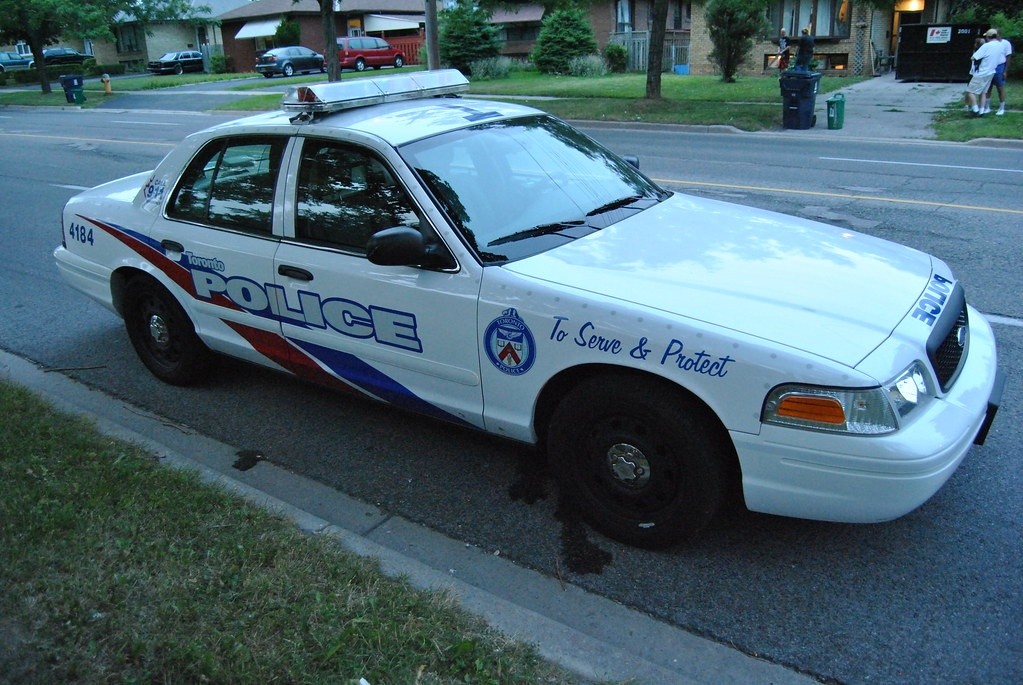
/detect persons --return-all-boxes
[778,28,792,73]
[795,29,814,71]
[963,28,1012,115]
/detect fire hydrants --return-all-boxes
[100,73,112,94]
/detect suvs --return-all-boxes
[42,48,94,65]
[0,51,38,72]
[322,36,406,72]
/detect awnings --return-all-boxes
[234,17,286,38]
[491,4,545,23]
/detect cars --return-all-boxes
[256,45,325,77]
[55,68,1008,552]
[147,50,203,75]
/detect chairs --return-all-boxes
[425,146,486,217]
[302,151,371,248]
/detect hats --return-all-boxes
[983,29,997,36]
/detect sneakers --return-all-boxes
[961,105,968,112]
[983,99,991,113]
[969,105,984,115]
[996,102,1005,115]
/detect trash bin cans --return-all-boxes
[779,70,822,130]
[71,89,84,105]
[825,93,845,130]
[59,75,87,102]
